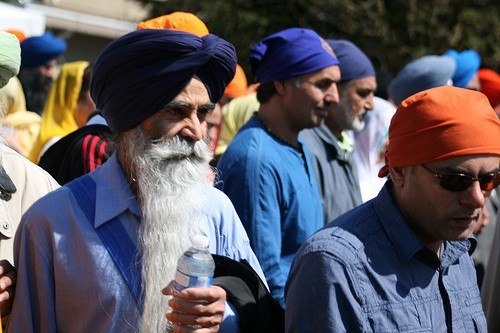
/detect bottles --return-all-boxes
[164,234,215,333]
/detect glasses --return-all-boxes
[419,162,500,192]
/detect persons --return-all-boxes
[297,38,377,228]
[214,28,341,313]
[0,10,500,175]
[284,85,500,333]
[0,33,62,333]
[7,30,283,333]
